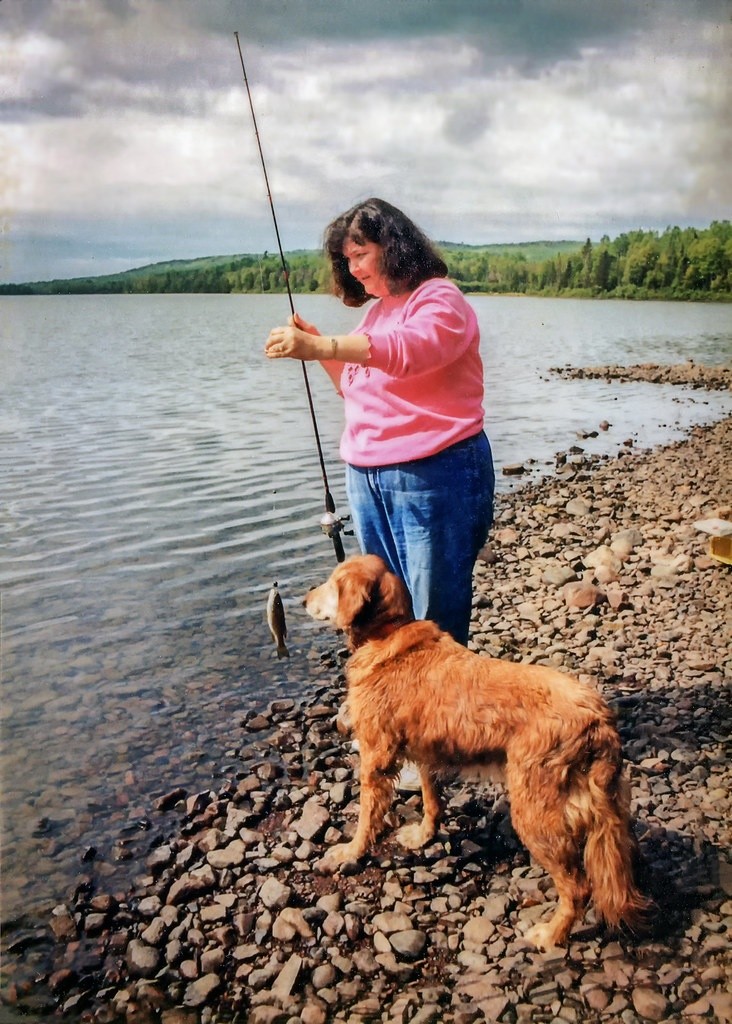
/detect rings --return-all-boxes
[270,344,283,352]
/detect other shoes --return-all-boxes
[388,758,442,791]
[352,739,360,751]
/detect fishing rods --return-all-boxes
[232,30,347,562]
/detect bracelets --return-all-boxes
[330,336,337,358]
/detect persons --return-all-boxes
[264,198,495,791]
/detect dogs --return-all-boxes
[301,554,663,948]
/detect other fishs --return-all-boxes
[267,588,290,660]
